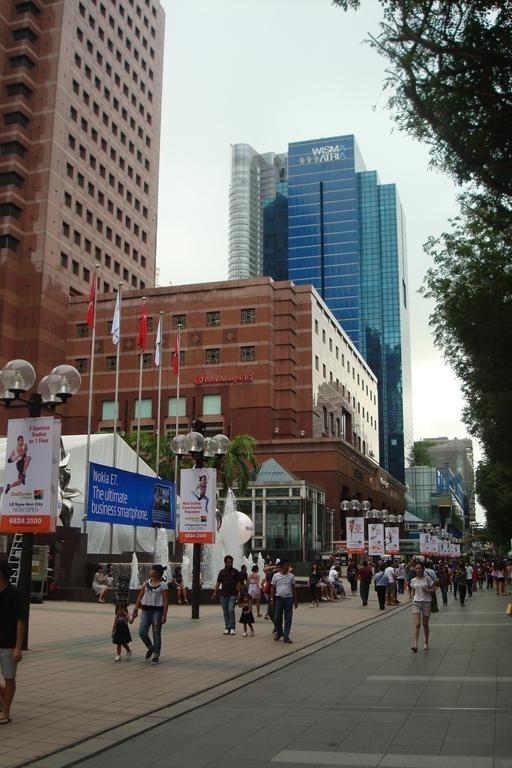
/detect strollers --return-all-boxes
[112,574,131,607]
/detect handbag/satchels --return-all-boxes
[431,589,439,613]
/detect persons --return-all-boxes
[191,470,209,513]
[308,553,511,613]
[0,558,29,725]
[5,433,33,494]
[404,562,436,652]
[211,555,298,644]
[172,566,188,604]
[92,564,114,603]
[348,519,356,539]
[112,564,169,662]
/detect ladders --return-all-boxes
[8,533,23,588]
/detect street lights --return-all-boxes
[416,522,462,561]
[340,498,371,572]
[381,513,404,566]
[363,508,388,573]
[171,419,232,620]
[0,355,82,650]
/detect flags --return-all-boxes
[86,267,97,328]
[172,330,180,375]
[136,303,148,353]
[111,287,121,345]
[154,315,164,366]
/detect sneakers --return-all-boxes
[273,632,292,643]
[144,646,159,665]
[19,473,25,484]
[5,483,9,494]
[114,650,131,661]
